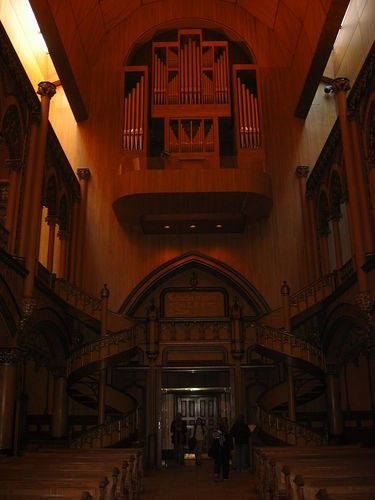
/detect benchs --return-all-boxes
[0,447,144,500]
[252,444,375,500]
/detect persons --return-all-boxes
[233,415,252,473]
[212,425,234,480]
[189,418,208,467]
[171,412,188,465]
